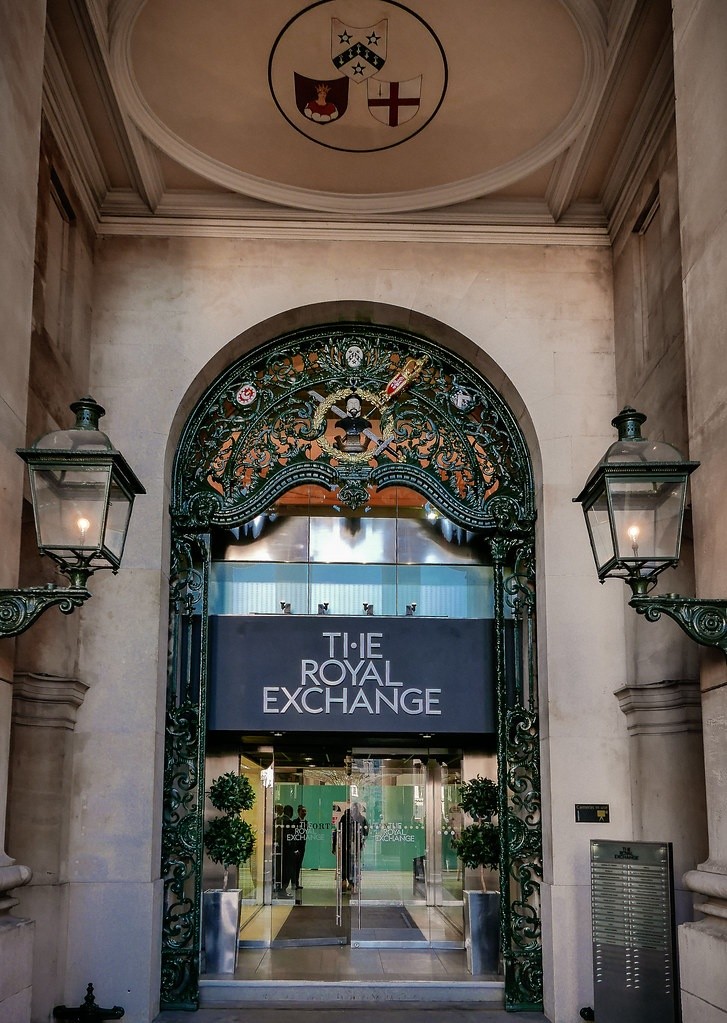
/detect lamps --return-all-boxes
[363,602,374,615]
[281,601,291,614]
[406,603,417,616]
[318,602,330,615]
[0,390,147,633]
[573,406,727,658]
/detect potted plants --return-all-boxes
[202,768,256,973]
[452,774,500,974]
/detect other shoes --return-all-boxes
[277,892,294,899]
[291,885,304,890]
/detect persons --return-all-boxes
[274,803,308,899]
[335,392,373,435]
[332,802,370,894]
